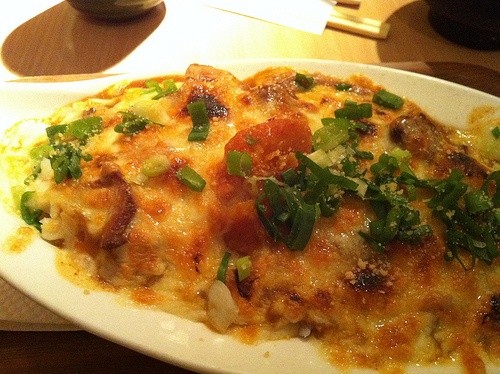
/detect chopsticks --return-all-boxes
[326,12,391,39]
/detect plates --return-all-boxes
[0,59,500,374]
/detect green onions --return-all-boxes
[15,79,500,271]
